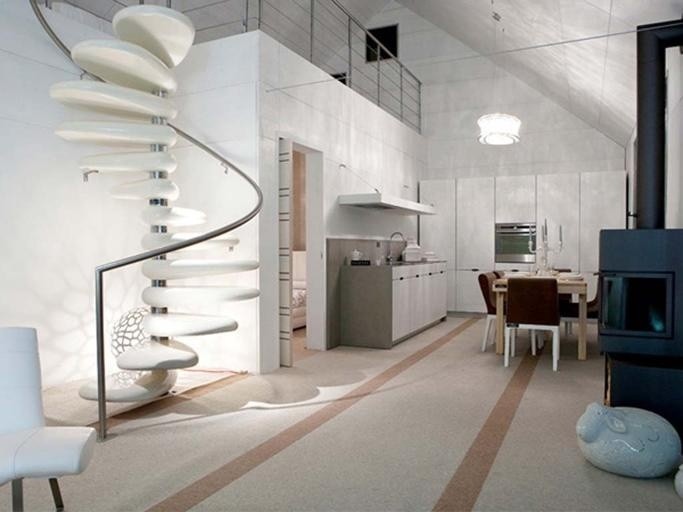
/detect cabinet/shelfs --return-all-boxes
[338,263,448,350]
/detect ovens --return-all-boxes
[495,221,537,264]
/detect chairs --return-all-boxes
[1,326,98,512]
[477,268,600,372]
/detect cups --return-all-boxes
[350,248,363,260]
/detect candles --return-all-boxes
[529,219,562,243]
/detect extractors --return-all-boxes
[335,193,438,216]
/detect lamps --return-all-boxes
[476,2,521,146]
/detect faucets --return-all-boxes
[387,231,408,263]
